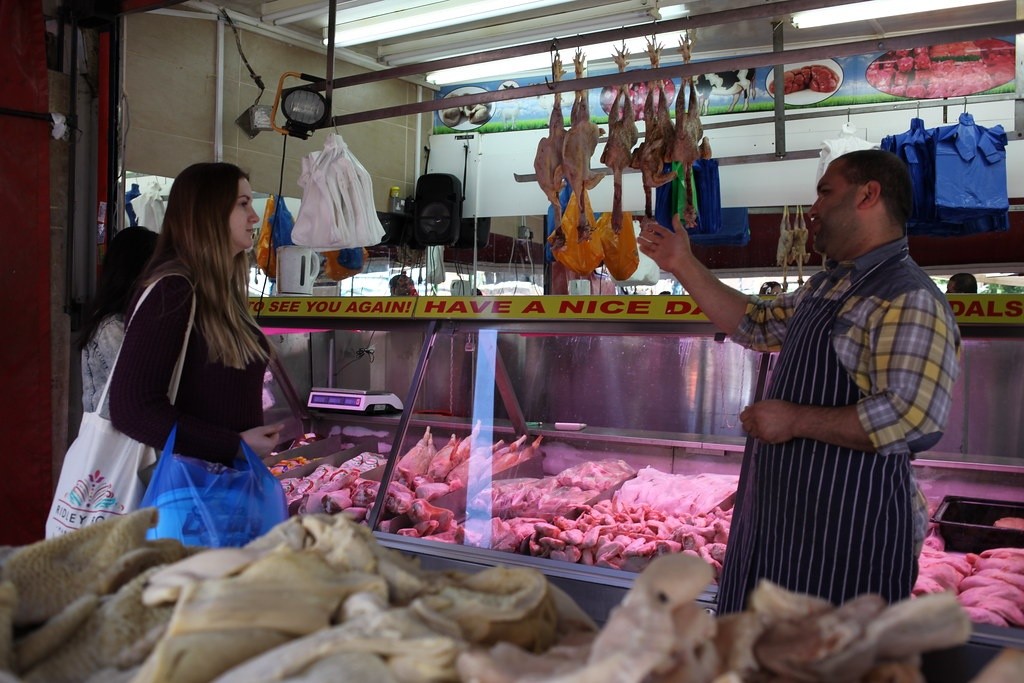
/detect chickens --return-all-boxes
[792,205,811,287]
[561,48,607,246]
[638,36,679,233]
[669,35,713,230]
[599,43,639,236]
[532,54,569,252]
[775,206,796,293]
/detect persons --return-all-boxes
[945,273,977,294]
[104,159,283,550]
[635,149,959,638]
[388,275,422,359]
[79,227,163,423]
[758,282,783,295]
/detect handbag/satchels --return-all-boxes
[139,424,288,547]
[44,275,196,540]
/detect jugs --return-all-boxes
[275,245,320,296]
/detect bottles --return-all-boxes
[390,186,401,212]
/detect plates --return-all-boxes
[765,59,844,105]
[438,86,496,131]
[498,81,520,102]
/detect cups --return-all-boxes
[568,279,590,296]
[450,280,471,296]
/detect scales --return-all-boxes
[307,387,404,415]
